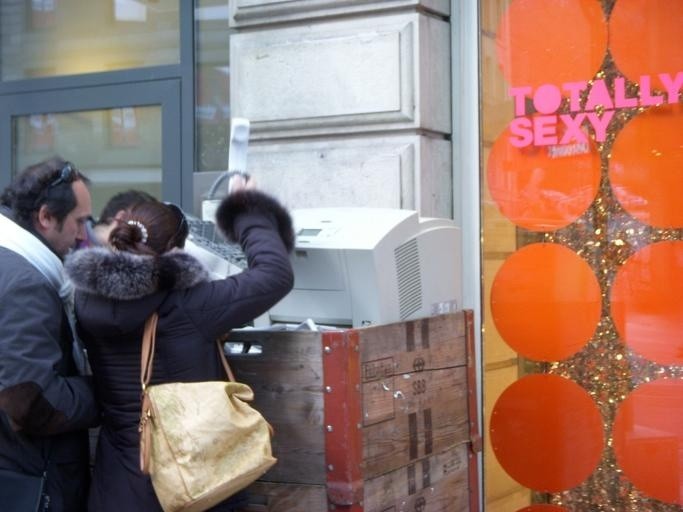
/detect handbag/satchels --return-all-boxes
[0,470,55,512]
[138,379,277,512]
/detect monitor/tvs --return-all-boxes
[270,206,423,326]
[419,217,464,316]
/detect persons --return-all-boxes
[75,174,297,511]
[75,190,156,248]
[0,157,103,512]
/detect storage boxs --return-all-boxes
[215,310,481,512]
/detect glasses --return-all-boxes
[168,202,190,240]
[50,160,75,189]
